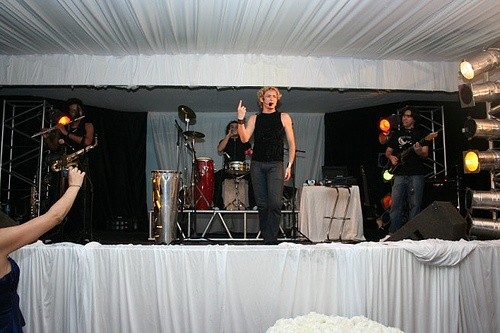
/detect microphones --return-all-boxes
[58,139,74,150]
[263,101,272,106]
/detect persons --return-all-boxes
[0,166,85,333]
[380,106,428,242]
[237,86,295,245]
[40,98,94,245]
[210,121,258,211]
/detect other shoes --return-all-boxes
[210,207,220,210]
[379,235,390,242]
[252,206,258,211]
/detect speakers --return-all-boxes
[383,201,467,241]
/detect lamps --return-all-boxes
[462,149,500,174]
[462,116,500,140]
[459,39,500,79]
[465,214,500,240]
[458,81,500,109]
[465,187,500,213]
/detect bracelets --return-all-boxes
[287,162,292,168]
[70,185,81,187]
[238,119,244,124]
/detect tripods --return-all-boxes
[224,177,250,210]
[186,134,210,210]
[283,148,312,243]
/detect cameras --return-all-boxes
[62,169,68,177]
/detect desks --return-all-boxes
[296,185,367,240]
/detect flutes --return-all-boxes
[30,115,85,138]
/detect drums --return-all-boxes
[192,157,215,210]
[223,160,252,173]
[150,169,183,246]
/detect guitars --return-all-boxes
[388,132,438,174]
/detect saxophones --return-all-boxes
[50,137,98,172]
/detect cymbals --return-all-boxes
[183,130,206,139]
[178,104,197,119]
